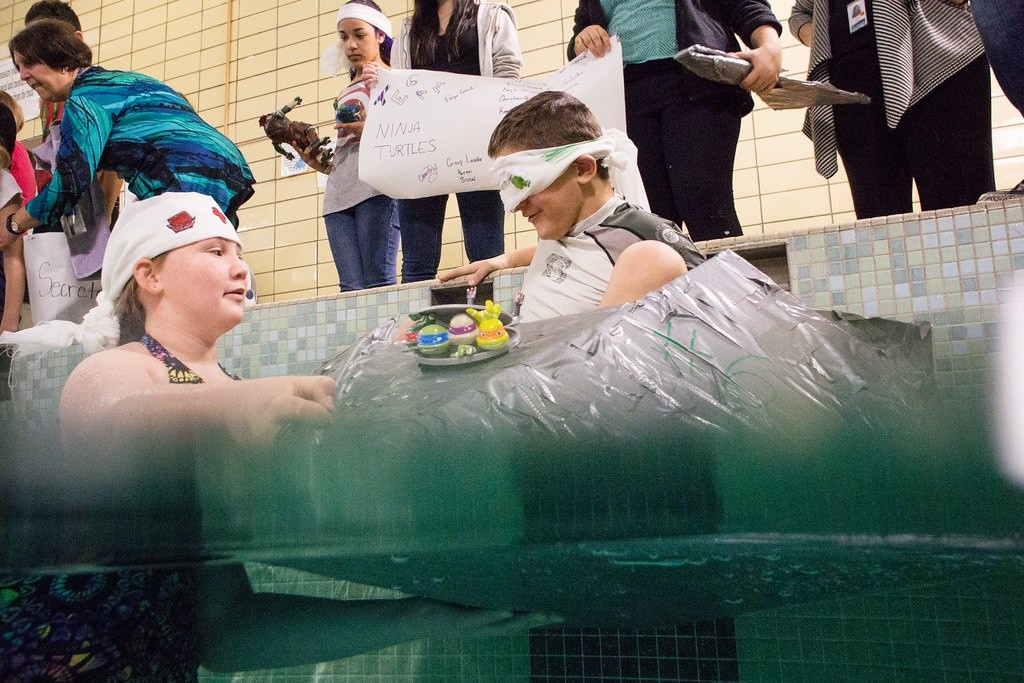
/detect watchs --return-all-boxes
[11,214,25,233]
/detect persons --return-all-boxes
[336,101,365,125]
[969,0,1024,205]
[0,89,39,334]
[433,90,708,325]
[0,17,257,253]
[290,0,402,292]
[567,0,784,243]
[361,0,523,285]
[787,0,998,221]
[258,95,334,164]
[1,191,568,682]
[23,0,121,232]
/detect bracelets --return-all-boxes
[6,213,24,235]
